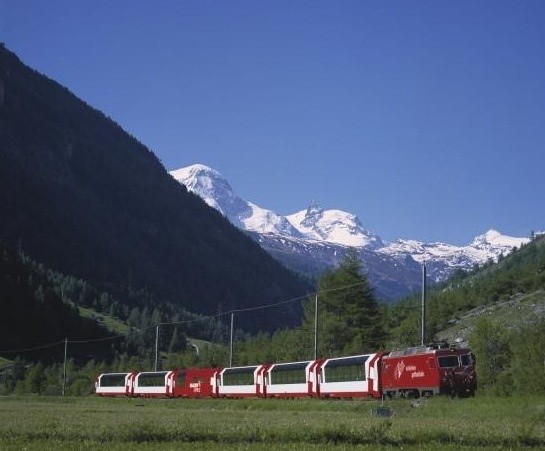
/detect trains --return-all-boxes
[94,342,477,403]
